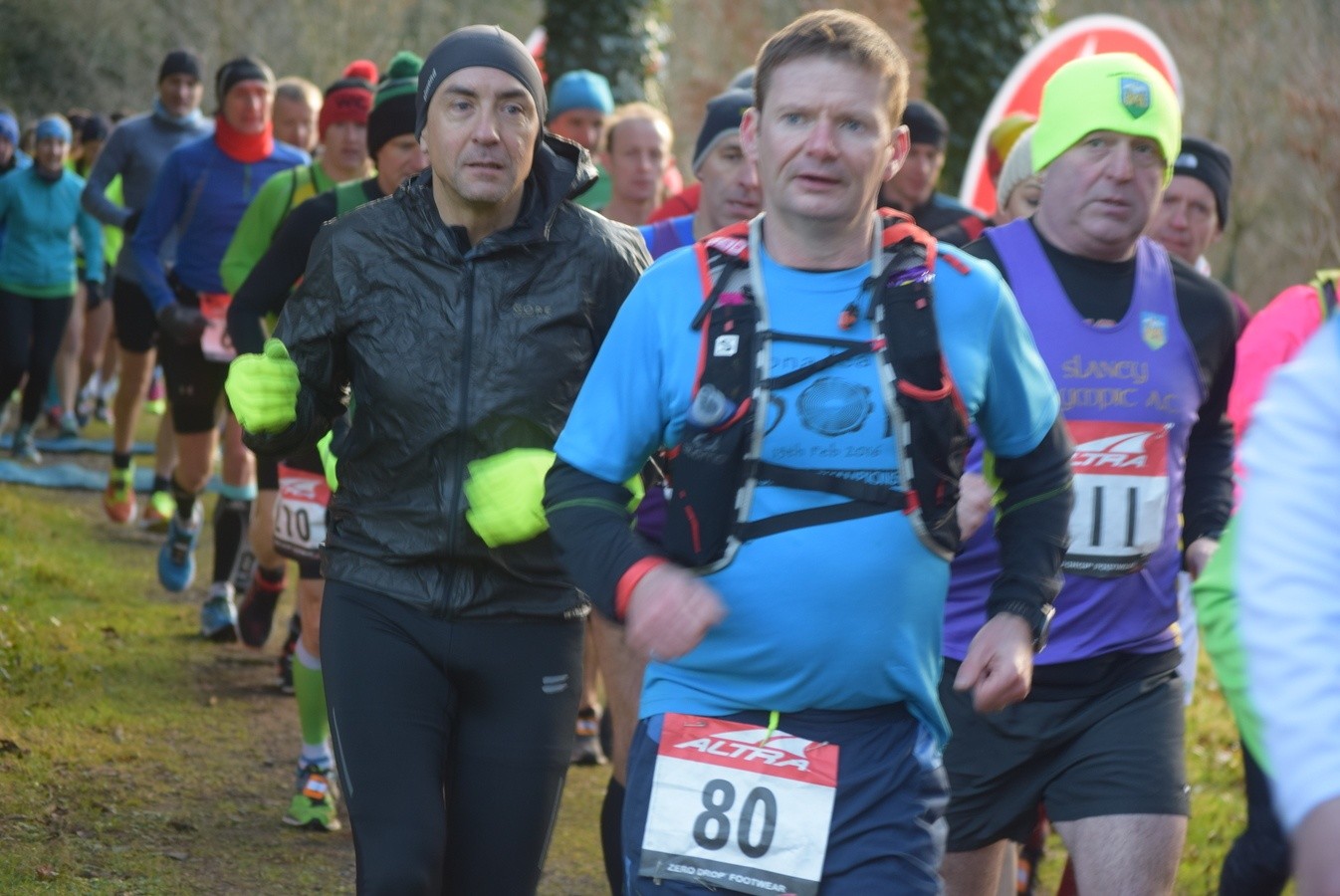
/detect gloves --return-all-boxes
[222,336,298,436]
[122,210,141,231]
[461,448,646,548]
[158,305,205,345]
[84,278,103,311]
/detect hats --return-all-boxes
[899,101,948,142]
[987,117,1032,216]
[1174,134,1232,233]
[362,49,425,160]
[215,58,271,113]
[0,111,114,147]
[692,62,754,173]
[318,60,379,144]
[414,24,548,138]
[1031,51,1182,191]
[157,52,204,85]
[544,69,615,127]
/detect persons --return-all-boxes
[951,19,1251,896]
[545,9,1080,896]
[225,22,659,896]
[0,8,1340,896]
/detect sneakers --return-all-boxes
[280,761,341,832]
[202,595,238,643]
[239,561,290,647]
[233,552,258,591]
[142,490,178,523]
[280,638,299,694]
[158,500,204,593]
[103,455,139,525]
[569,717,609,765]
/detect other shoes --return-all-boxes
[0,375,166,467]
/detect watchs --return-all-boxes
[995,596,1056,656]
